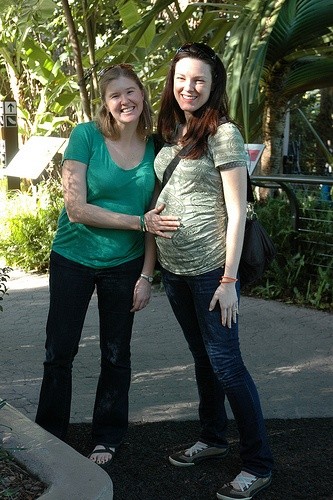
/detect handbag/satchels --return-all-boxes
[238,213,274,281]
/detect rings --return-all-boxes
[232,309,237,312]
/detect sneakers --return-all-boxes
[216,470,272,500]
[169,442,229,466]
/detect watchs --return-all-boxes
[140,274,153,284]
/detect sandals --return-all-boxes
[87,443,116,468]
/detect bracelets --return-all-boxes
[141,213,149,232]
[220,276,238,284]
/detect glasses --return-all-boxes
[101,63,134,76]
[176,41,216,66]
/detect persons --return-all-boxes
[33,63,181,464]
[153,42,274,500]
